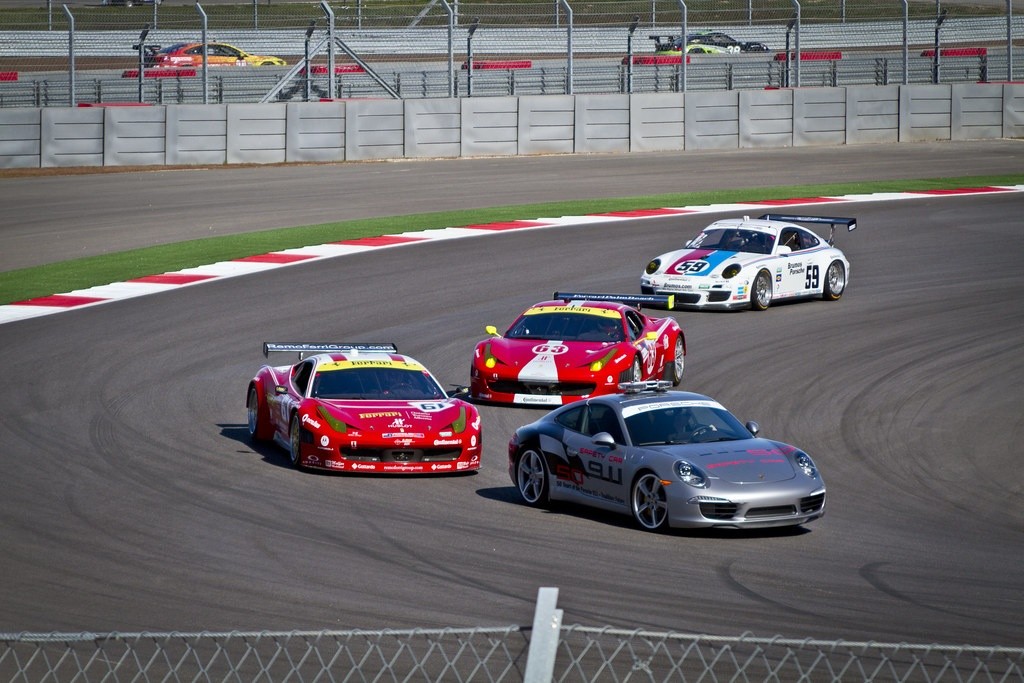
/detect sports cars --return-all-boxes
[639,213,857,311]
[468,291,688,406]
[506,378,827,531]
[243,339,485,473]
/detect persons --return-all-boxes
[662,408,717,441]
[596,317,618,334]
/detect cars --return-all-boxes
[650,30,770,54]
[132,42,287,68]
